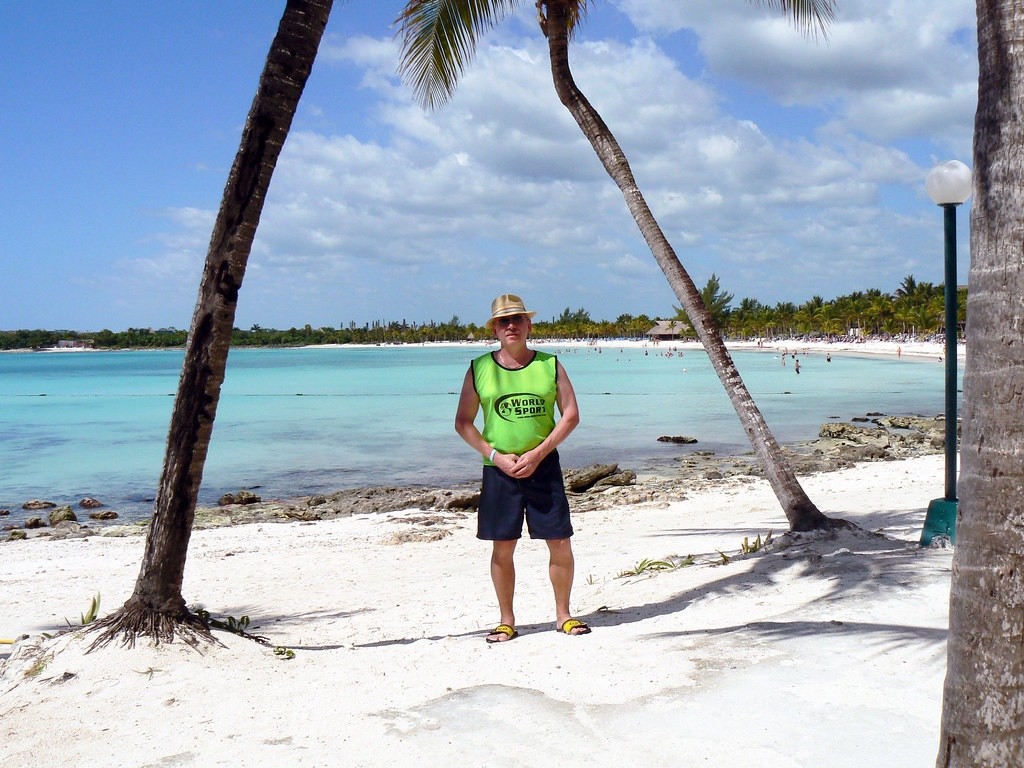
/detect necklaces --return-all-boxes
[496,352,530,370]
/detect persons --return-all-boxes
[896,347,902,357]
[383,329,966,375]
[794,360,801,374]
[454,293,593,643]
[826,354,832,362]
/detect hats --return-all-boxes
[486,294,538,328]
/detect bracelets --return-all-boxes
[489,449,497,462]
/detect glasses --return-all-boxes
[498,315,527,327]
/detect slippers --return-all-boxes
[556,618,591,635]
[486,624,518,643]
